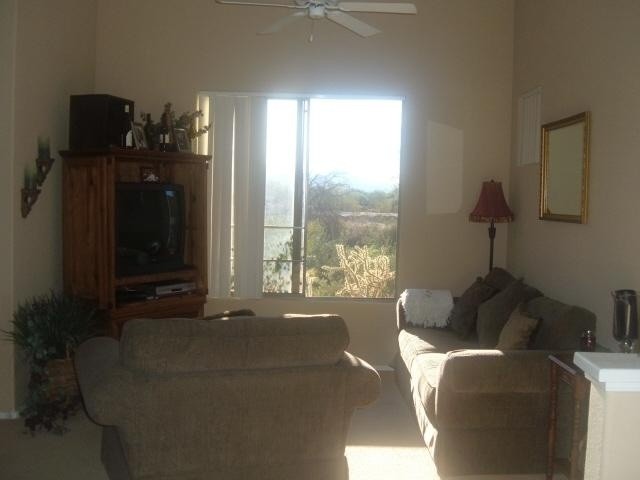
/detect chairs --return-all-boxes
[77,314,382,480]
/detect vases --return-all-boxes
[44,359,82,405]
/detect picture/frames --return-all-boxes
[130,121,148,150]
[173,127,191,153]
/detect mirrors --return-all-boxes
[539,111,590,224]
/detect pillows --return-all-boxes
[477,278,523,349]
[496,302,541,349]
[448,276,497,342]
[203,309,256,320]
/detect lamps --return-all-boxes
[469,179,514,274]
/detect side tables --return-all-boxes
[546,350,591,480]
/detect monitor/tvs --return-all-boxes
[116,181,187,278]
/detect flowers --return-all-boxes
[1,290,112,435]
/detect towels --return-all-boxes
[400,288,455,328]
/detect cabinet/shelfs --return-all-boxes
[60,148,211,340]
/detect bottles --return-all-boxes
[143,112,171,153]
[611,289,638,342]
[580,329,596,352]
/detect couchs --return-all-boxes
[394,267,596,478]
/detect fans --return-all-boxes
[216,0,418,38]
